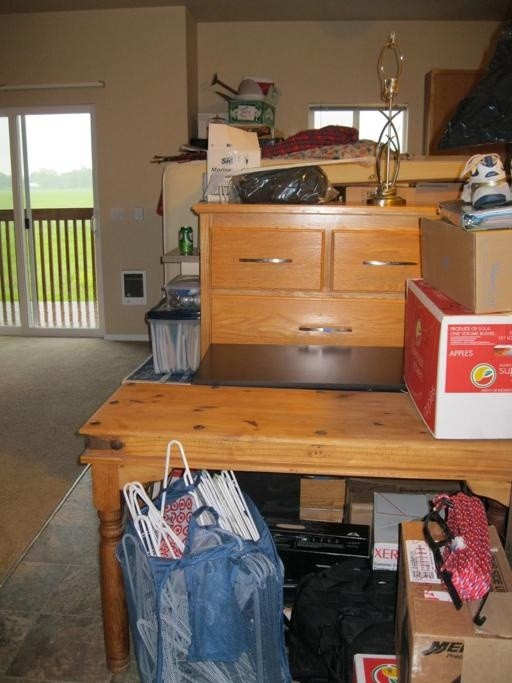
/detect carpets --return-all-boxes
[0,334,152,590]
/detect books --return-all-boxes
[151,322,200,373]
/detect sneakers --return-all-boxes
[458,154,512,209]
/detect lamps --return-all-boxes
[211,72,267,100]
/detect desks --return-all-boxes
[190,342,408,394]
[76,384,512,683]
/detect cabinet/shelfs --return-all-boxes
[189,203,442,359]
[161,159,207,300]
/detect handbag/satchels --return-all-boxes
[287,556,398,682]
[424,492,493,611]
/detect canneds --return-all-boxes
[178,225,193,255]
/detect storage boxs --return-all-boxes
[207,123,262,202]
[162,275,200,311]
[401,278,512,440]
[393,520,511,683]
[345,182,464,205]
[418,215,512,313]
[228,98,276,128]
[352,653,397,683]
[145,299,201,376]
[368,492,430,572]
